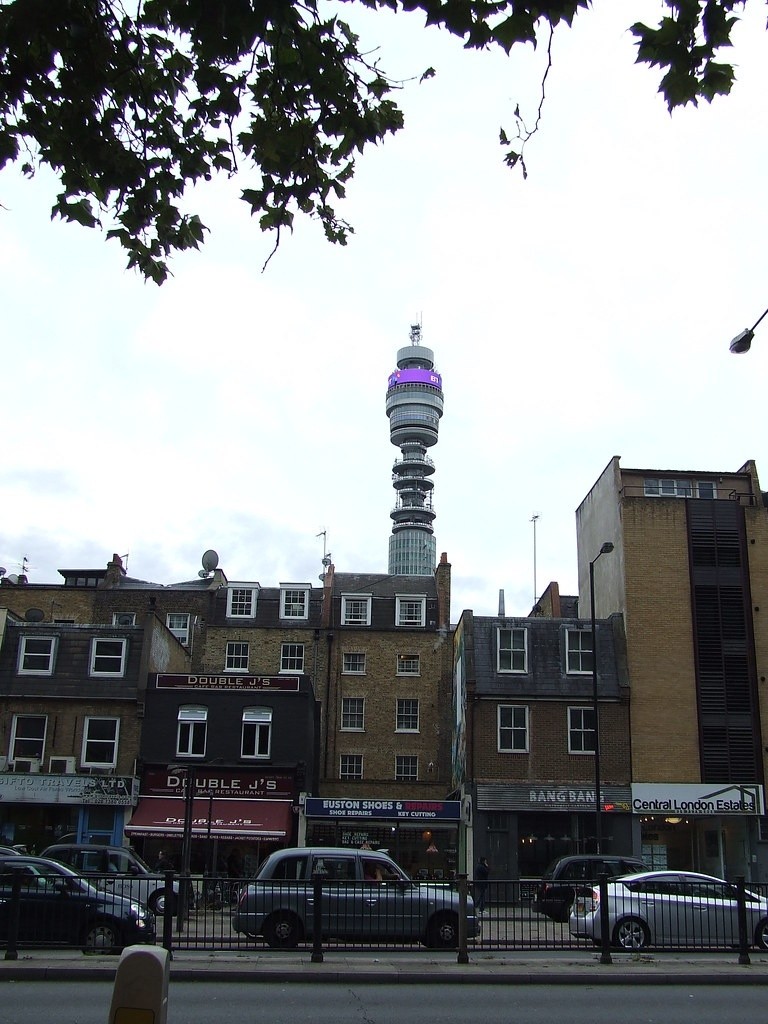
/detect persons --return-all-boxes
[360,840,372,850]
[475,857,490,914]
[108,855,119,871]
[155,849,170,874]
[227,848,243,878]
[364,861,399,880]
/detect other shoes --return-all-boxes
[479,911,489,915]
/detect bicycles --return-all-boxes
[204,877,239,910]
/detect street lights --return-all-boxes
[590,541,615,854]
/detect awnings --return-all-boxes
[123,797,292,845]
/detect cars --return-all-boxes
[0,855,156,955]
[569,870,768,952]
[0,845,66,890]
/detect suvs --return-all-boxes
[55,830,113,845]
[233,846,480,951]
[38,844,196,916]
[532,855,652,923]
